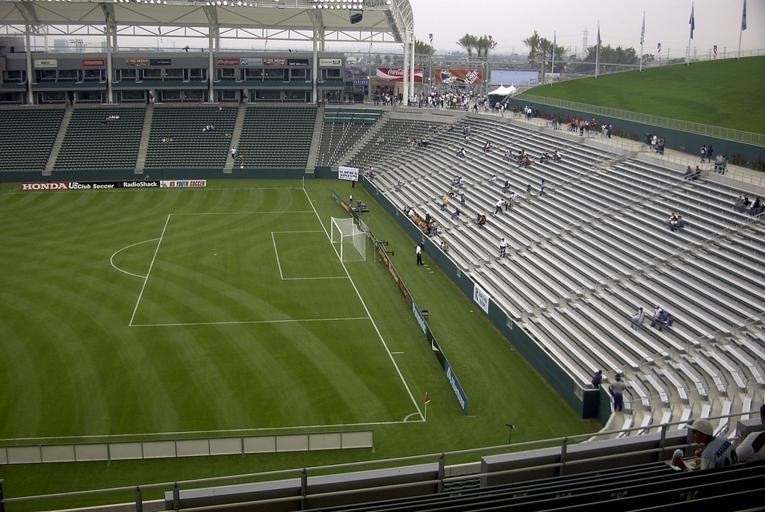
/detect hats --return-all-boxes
[685,420,714,438]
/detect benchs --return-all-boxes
[342,104,765,511]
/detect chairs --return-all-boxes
[2,104,346,179]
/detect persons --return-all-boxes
[735,403,765,461]
[671,419,738,472]
[369,123,563,168]
[105,90,248,143]
[551,113,613,139]
[632,305,673,331]
[608,379,623,397]
[684,143,728,181]
[394,176,546,267]
[667,213,684,232]
[318,85,489,115]
[592,368,604,389]
[231,147,237,159]
[490,96,534,121]
[729,193,765,216]
[610,374,626,412]
[645,130,665,155]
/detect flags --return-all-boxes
[688,3,695,39]
[742,0,747,31]
[552,31,557,50]
[424,391,432,404]
[640,13,645,45]
[597,23,601,47]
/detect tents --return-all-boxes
[488,85,516,108]
